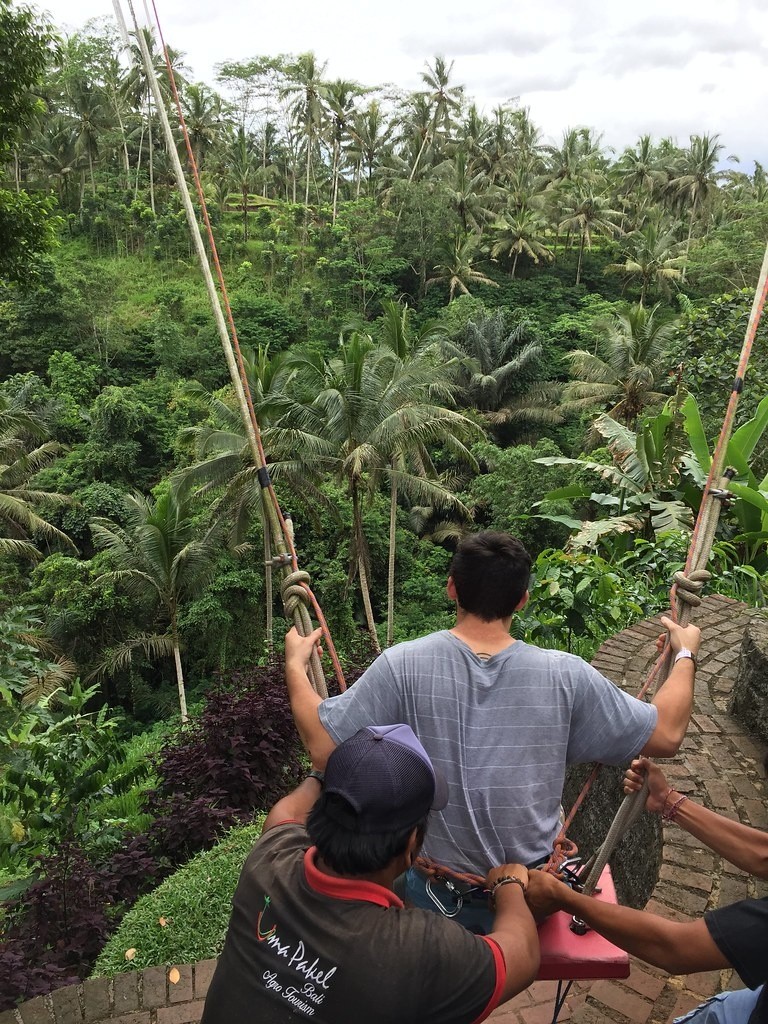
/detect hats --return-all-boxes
[320,724,450,834]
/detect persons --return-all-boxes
[285,531,701,937]
[198,724,541,1024]
[524,758,767,1024]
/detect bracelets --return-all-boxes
[662,788,687,820]
[675,649,697,673]
[307,770,324,785]
[483,875,526,897]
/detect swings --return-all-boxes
[115,0,766,983]
